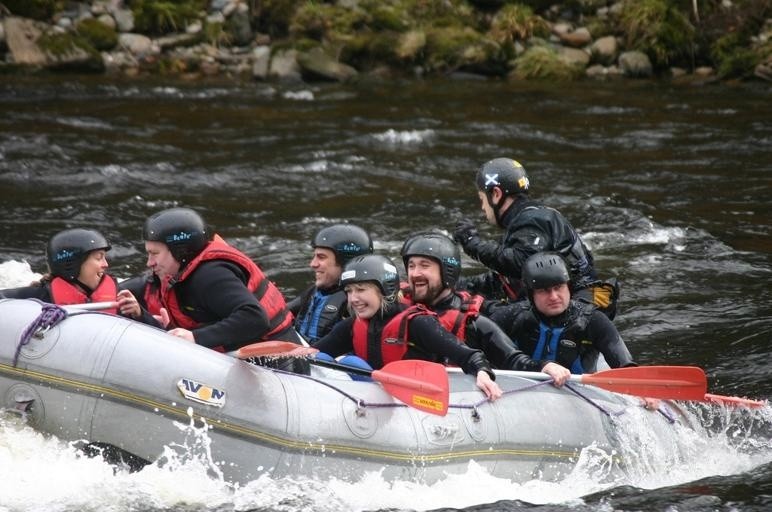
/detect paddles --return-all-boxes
[223,341,319,369]
[446,362,707,406]
[309,358,449,419]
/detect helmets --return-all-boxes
[521,251,571,290]
[400,224,462,288]
[340,253,400,299]
[476,156,531,193]
[315,223,374,264]
[143,206,208,262]
[46,227,112,282]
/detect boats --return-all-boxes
[0,275,705,492]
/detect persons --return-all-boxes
[490,251,660,411]
[141,207,311,375]
[395,233,572,388]
[455,158,619,320]
[311,253,504,401]
[284,224,373,346]
[0,229,160,326]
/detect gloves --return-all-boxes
[455,218,479,242]
